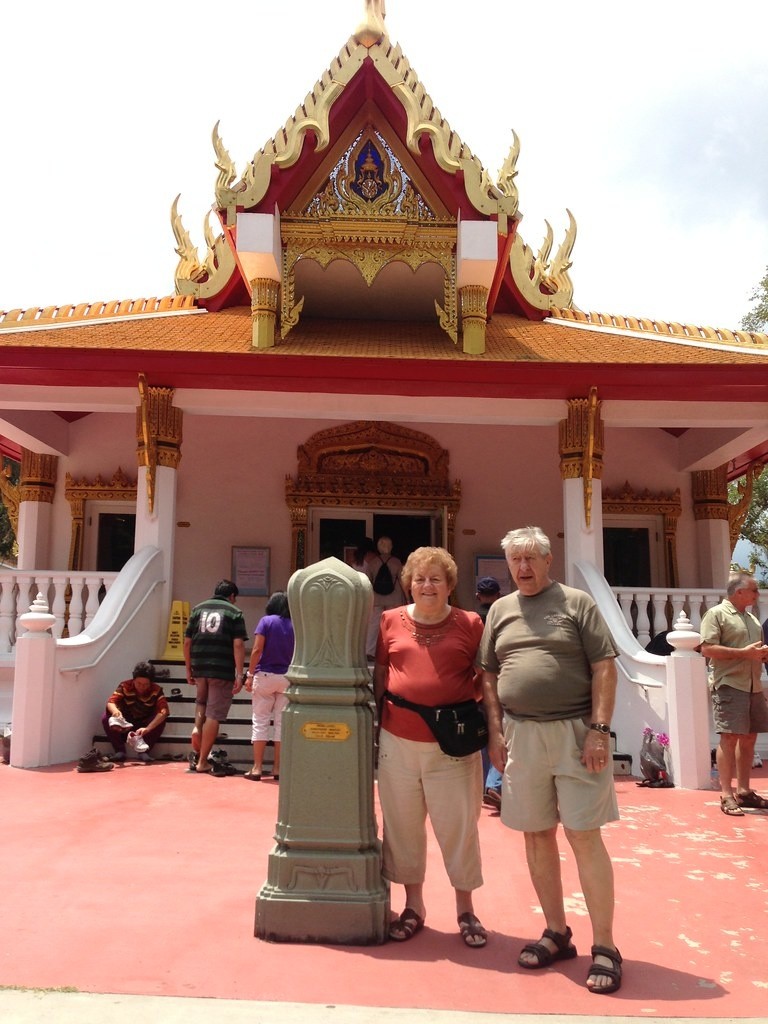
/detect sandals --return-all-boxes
[736,792,768,808]
[519,927,577,969]
[587,945,623,992]
[720,796,744,816]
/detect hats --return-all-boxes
[477,577,500,593]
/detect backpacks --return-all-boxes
[374,555,397,595]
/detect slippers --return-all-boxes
[158,752,188,761]
[458,912,487,947]
[483,794,501,811]
[389,908,424,941]
[274,775,279,780]
[244,769,261,781]
[635,779,675,788]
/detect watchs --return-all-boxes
[246,671,253,677]
[590,723,611,735]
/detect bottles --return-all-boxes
[710,764,720,789]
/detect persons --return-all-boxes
[700,574,768,815]
[102,662,171,762]
[245,593,296,782]
[476,528,624,995]
[351,536,505,806]
[183,580,244,771]
[373,548,487,947]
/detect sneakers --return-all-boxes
[207,749,229,764]
[126,731,149,753]
[77,748,114,772]
[108,716,133,732]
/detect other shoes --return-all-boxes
[207,762,236,776]
[751,752,762,768]
[367,654,375,662]
[216,732,228,739]
[187,750,199,770]
[171,688,183,698]
[154,668,170,678]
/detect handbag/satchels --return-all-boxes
[420,699,488,757]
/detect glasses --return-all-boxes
[752,588,758,593]
